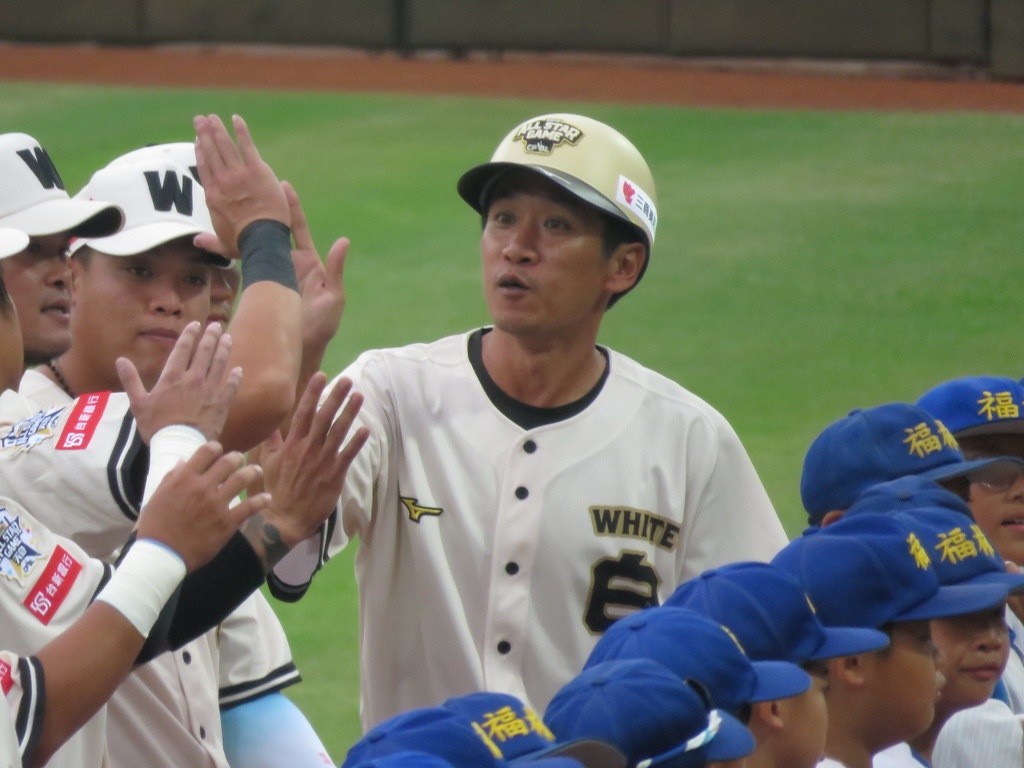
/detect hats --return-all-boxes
[132,142,204,188]
[342,707,586,768]
[442,692,627,768]
[583,605,810,708]
[883,508,1024,596]
[1,131,125,240]
[354,751,453,768]
[541,657,756,768]
[845,474,977,522]
[770,512,1009,631]
[916,376,1024,438]
[0,224,30,261]
[801,403,1023,516]
[63,152,237,271]
[659,562,891,666]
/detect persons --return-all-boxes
[341,366,1024,768]
[284,110,796,743]
[0,112,372,767]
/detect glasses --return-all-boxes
[972,461,1020,492]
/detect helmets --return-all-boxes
[459,114,656,309]
